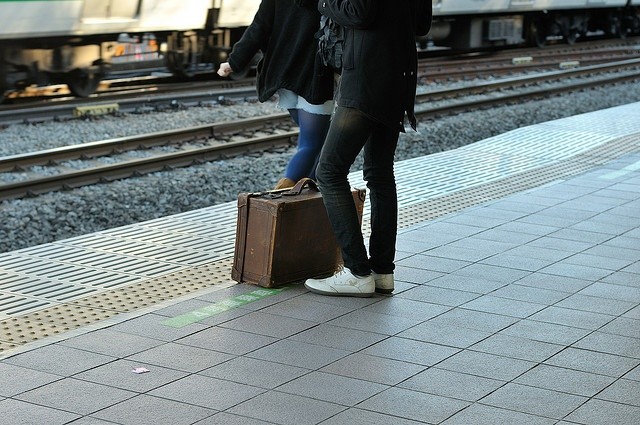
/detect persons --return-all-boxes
[216,0,338,193]
[303,0,432,298]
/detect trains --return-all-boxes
[0,0,640,105]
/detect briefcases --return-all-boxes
[231,178,366,289]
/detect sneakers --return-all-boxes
[371,269,394,293]
[304,264,376,297]
